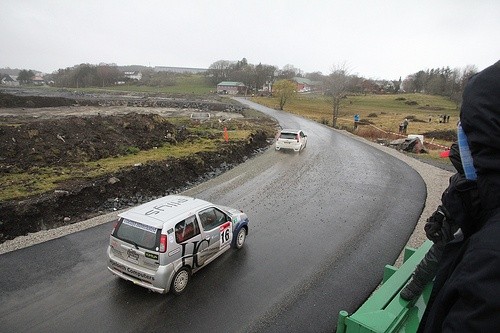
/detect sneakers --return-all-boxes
[401,282,424,300]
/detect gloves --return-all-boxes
[424,208,444,237]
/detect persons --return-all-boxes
[400,141,471,302]
[354,112,359,128]
[416,58,500,333]
[428,117,432,122]
[399,118,409,134]
[439,114,450,123]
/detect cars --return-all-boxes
[274,129,307,154]
[106,192,250,295]
